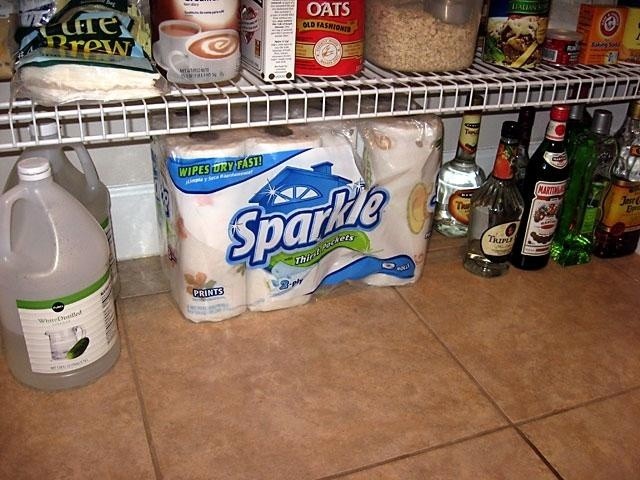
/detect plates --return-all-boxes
[152,39,170,73]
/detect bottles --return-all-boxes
[431,89,640,275]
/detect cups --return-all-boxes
[169,28,242,81]
[159,20,202,63]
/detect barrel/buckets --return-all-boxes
[0,157,121,392]
[4,122,121,302]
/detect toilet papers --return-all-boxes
[150,88,445,323]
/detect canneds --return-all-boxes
[474,0,550,69]
[542,28,584,66]
[295,0,367,76]
